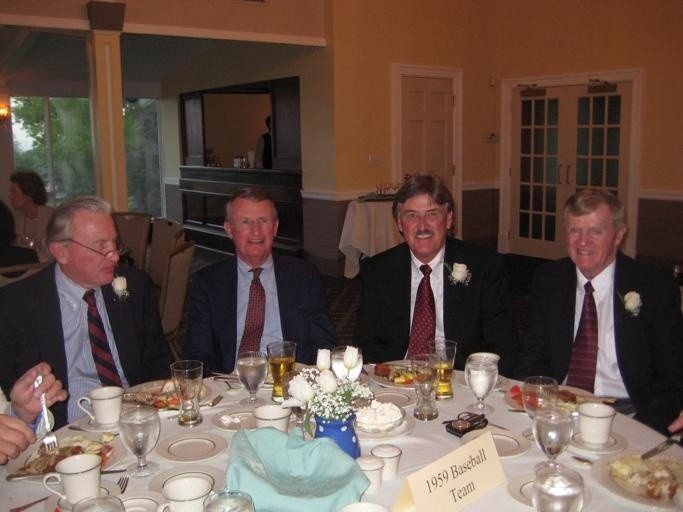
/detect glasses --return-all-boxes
[68,239,127,259]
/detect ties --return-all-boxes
[83,289,122,389]
[406,264,434,361]
[236,268,265,358]
[565,281,597,393]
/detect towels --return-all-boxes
[221,423,371,512]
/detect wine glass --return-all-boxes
[466,356,497,418]
[236,352,268,408]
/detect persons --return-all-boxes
[667,408,683,440]
[181,186,340,379]
[254,115,273,170]
[0,195,181,432]
[511,187,683,438]
[0,199,41,280]
[1,171,57,263]
[0,361,68,465]
[353,174,518,378]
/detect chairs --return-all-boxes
[0,258,54,285]
[144,218,185,285]
[109,211,151,276]
[148,241,196,362]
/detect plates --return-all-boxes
[6,360,683,512]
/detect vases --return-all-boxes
[313,408,362,458]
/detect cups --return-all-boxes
[470,352,502,365]
[427,339,457,401]
[266,341,297,404]
[411,355,442,421]
[332,346,363,382]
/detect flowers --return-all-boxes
[279,345,377,432]
[616,287,645,318]
[110,276,131,304]
[440,259,474,288]
